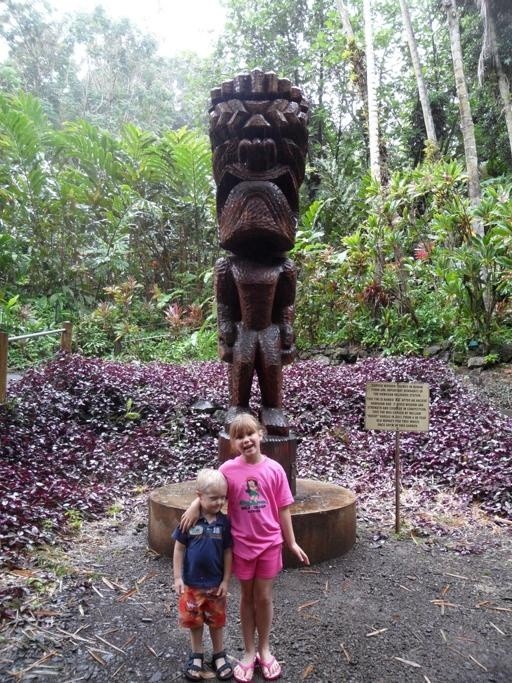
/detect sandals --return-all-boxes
[185,652,204,680]
[212,650,233,681]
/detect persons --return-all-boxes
[171,466,235,682]
[177,410,313,683]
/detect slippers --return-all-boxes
[234,653,256,682]
[257,651,282,680]
